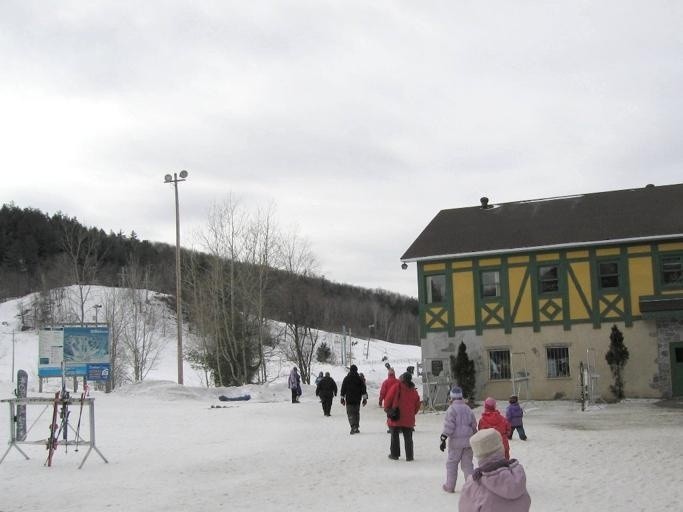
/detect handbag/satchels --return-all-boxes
[386,407,400,421]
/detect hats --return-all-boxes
[450,386,463,400]
[399,372,412,382]
[484,397,496,411]
[508,395,518,404]
[469,428,507,470]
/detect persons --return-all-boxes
[505,396,528,441]
[288,363,422,461]
[478,397,512,460]
[458,428,531,512]
[439,387,478,493]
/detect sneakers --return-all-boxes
[292,401,415,434]
[442,483,455,494]
[388,454,399,460]
[507,435,527,441]
[406,457,414,461]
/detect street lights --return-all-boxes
[367,324,375,359]
[91,304,102,324]
[162,167,193,386]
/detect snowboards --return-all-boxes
[15,369,27,441]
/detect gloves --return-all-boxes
[439,434,447,453]
[340,398,345,406]
[362,399,367,406]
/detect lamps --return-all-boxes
[401,261,408,273]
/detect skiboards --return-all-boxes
[61,361,69,453]
[47,391,59,466]
